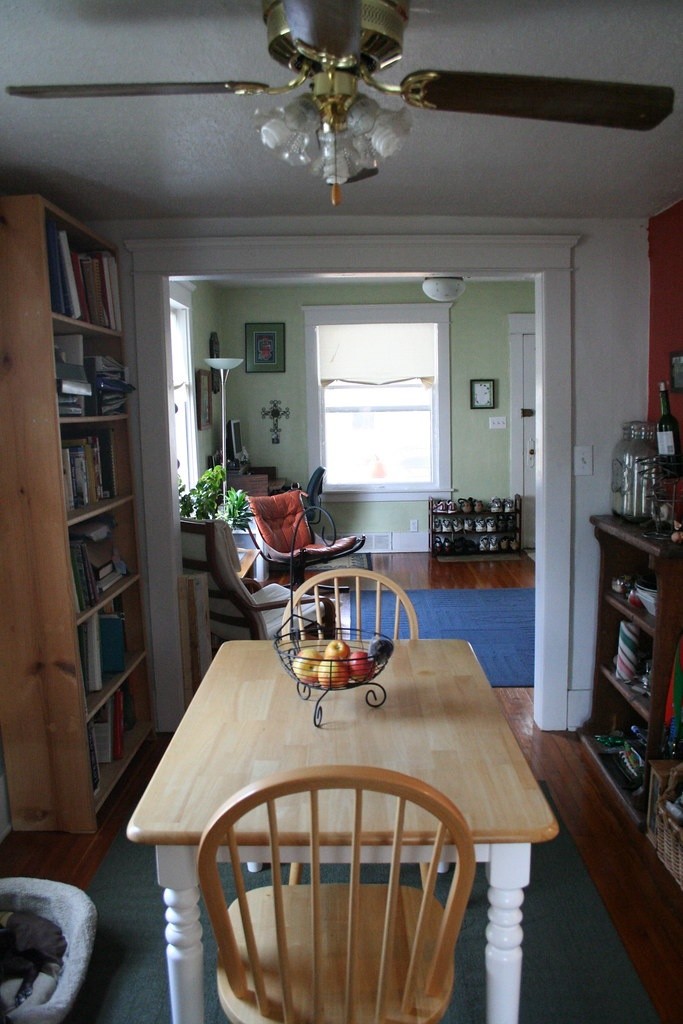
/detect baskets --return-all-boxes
[654,761,683,888]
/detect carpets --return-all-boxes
[61,780,661,1024]
[350,587,535,687]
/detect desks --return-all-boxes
[236,547,261,594]
[126,639,559,1024]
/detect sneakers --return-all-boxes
[432,495,519,552]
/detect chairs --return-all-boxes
[197,765,476,1024]
[303,466,325,525]
[245,489,366,591]
[281,567,429,886]
[180,517,336,651]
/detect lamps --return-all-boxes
[422,277,465,302]
[259,67,412,208]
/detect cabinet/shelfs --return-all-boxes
[428,493,522,557]
[0,192,156,836]
[575,513,683,833]
[220,467,286,496]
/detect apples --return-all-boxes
[292,639,374,688]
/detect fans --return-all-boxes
[5,1,674,131]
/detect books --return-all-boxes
[46,220,137,794]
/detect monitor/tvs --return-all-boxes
[225,419,245,468]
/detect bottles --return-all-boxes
[656,380,683,481]
[611,421,656,521]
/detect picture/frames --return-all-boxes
[195,369,213,429]
[244,323,285,373]
[670,352,683,393]
[469,379,495,409]
